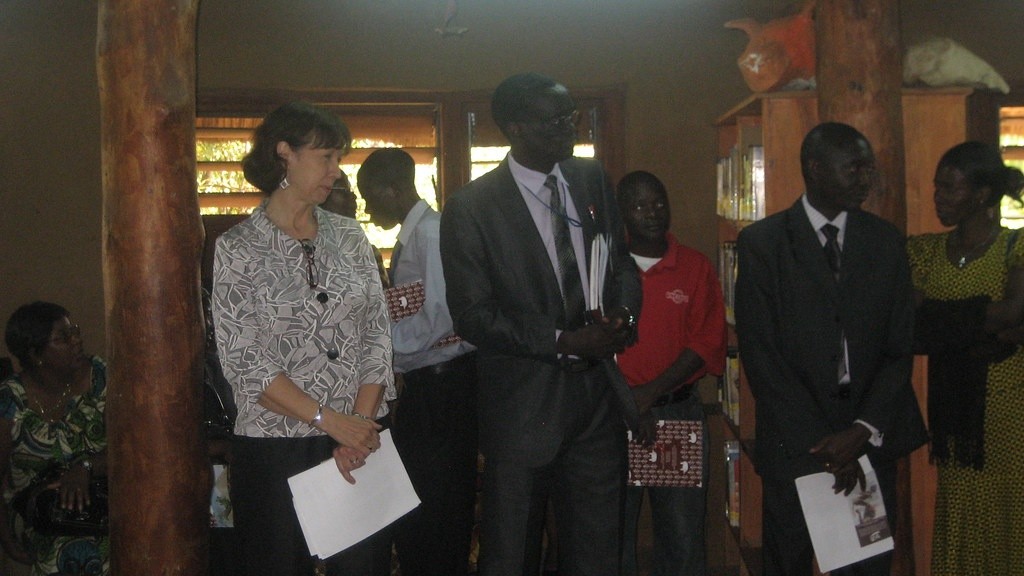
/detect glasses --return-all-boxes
[632,197,669,214]
[35,324,81,346]
[521,108,580,131]
[298,239,319,289]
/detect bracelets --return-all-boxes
[353,413,371,419]
[80,458,93,474]
[620,305,634,329]
[308,403,324,426]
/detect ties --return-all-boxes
[544,175,587,319]
[820,224,848,382]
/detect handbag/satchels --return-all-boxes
[13,462,108,536]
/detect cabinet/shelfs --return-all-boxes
[715,87,975,576]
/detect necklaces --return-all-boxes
[950,222,998,267]
[25,380,70,422]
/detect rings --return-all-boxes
[77,488,82,492]
[352,457,359,465]
[826,462,830,469]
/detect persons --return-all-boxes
[201,288,237,576]
[0,301,110,575]
[906,144,1024,576]
[320,170,390,298]
[735,122,928,576]
[440,71,643,576]
[617,171,727,576]
[357,149,477,576]
[213,102,396,576]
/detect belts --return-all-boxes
[653,382,693,405]
[403,352,473,384]
